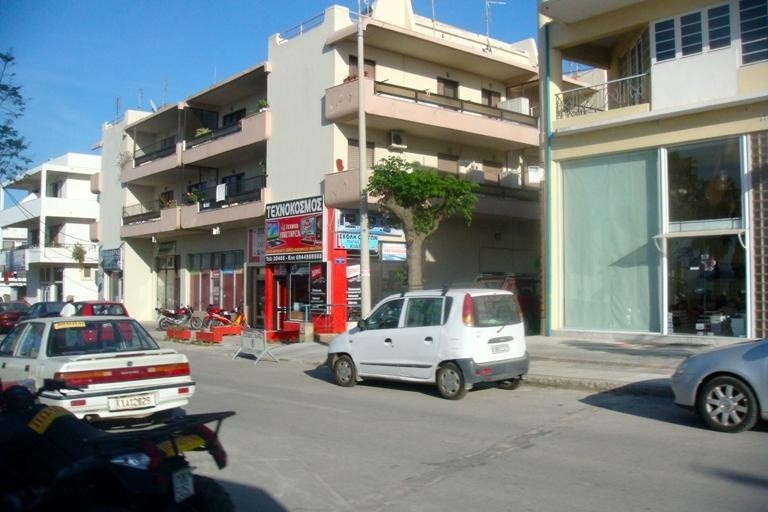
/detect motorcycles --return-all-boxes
[154,302,251,331]
[0,378,238,511]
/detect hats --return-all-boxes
[66,295,74,302]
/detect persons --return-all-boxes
[59,295,76,317]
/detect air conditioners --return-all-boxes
[388,130,407,149]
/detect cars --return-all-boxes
[669,336,768,434]
[1,296,196,421]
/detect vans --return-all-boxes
[324,288,529,400]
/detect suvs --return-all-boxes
[473,273,542,336]
